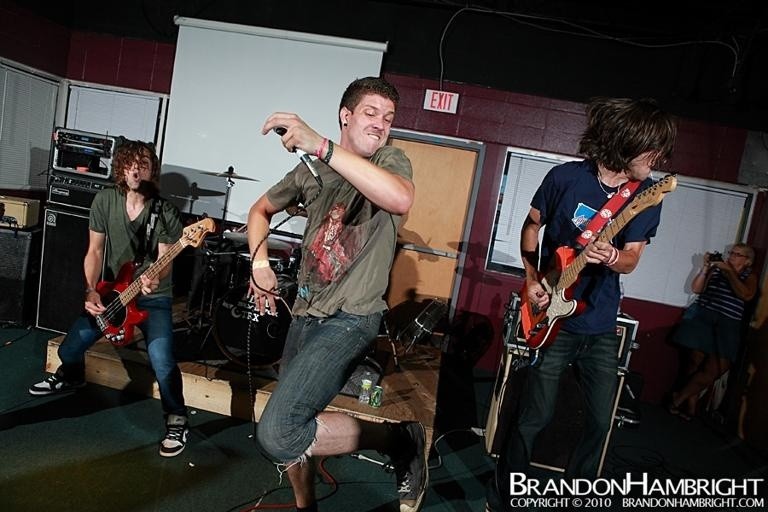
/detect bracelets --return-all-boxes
[603,246,616,266]
[606,248,621,269]
[82,286,95,294]
[314,138,327,158]
[697,268,706,279]
[323,140,334,166]
[251,259,270,271]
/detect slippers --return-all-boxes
[669,390,697,421]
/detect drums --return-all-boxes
[177,232,295,369]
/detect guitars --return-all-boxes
[95,219,216,346]
[520,172,681,350]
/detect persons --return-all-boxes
[245,73,431,510]
[475,94,681,510]
[665,240,760,423]
[22,140,192,461]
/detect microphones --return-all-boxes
[273,126,325,188]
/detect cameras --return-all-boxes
[710,252,722,261]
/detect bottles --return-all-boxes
[359,372,372,406]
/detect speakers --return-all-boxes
[486,349,625,479]
[33,209,107,336]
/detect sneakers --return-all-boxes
[160,414,190,457]
[28,375,86,395]
[379,421,429,512]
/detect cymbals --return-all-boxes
[172,184,224,197]
[204,169,257,182]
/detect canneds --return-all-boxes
[370,385,383,408]
[358,379,372,405]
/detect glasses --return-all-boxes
[728,251,748,257]
